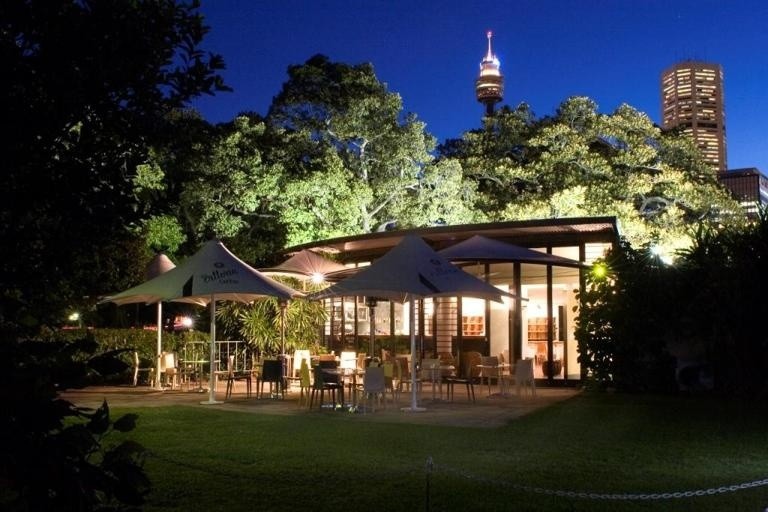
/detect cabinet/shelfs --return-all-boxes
[527,317,558,340]
[461,314,482,336]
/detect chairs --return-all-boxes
[417,352,539,407]
[134,352,210,393]
[215,351,410,415]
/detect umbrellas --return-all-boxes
[95,238,309,405]
[91,253,206,391]
[432,235,593,355]
[306,231,529,414]
[252,248,350,281]
